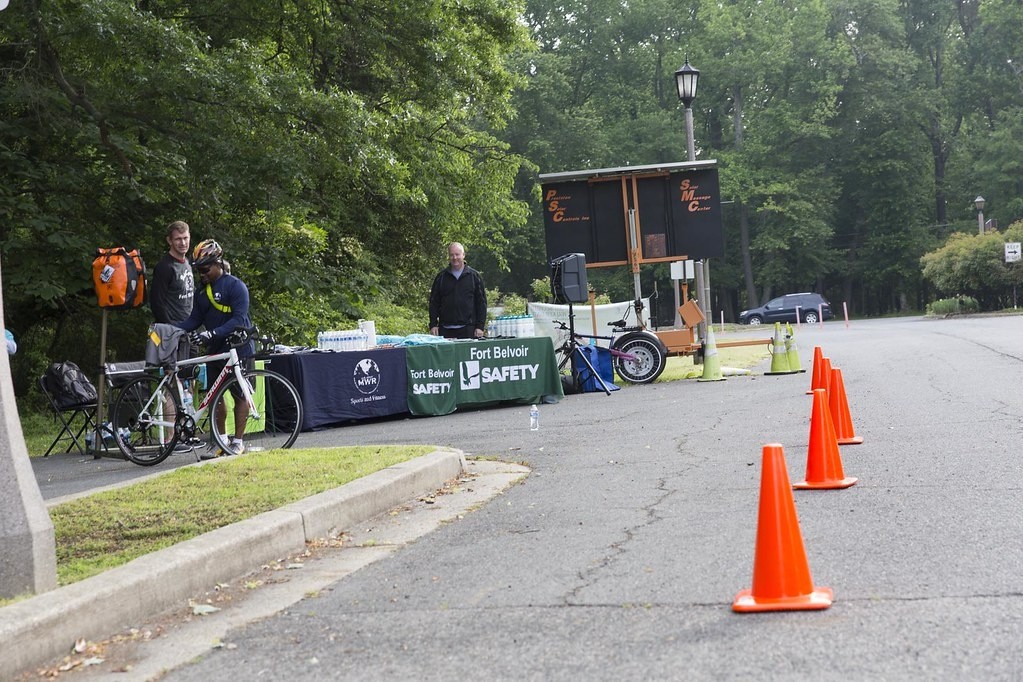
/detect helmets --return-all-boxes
[190,239,223,267]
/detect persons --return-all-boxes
[145,222,206,453]
[173,239,256,459]
[429,242,487,339]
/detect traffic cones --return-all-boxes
[793,388,858,489]
[764,322,798,375]
[824,368,863,444]
[784,321,807,373]
[805,347,832,394]
[732,444,835,612]
[721,366,751,376]
[698,326,727,381]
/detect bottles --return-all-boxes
[183,388,196,417]
[317,329,369,352]
[85,427,130,450]
[530,404,539,431]
[487,315,536,339]
[261,335,269,351]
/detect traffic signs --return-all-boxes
[1004,243,1021,262]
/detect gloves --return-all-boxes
[189,329,214,346]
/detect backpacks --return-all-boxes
[43,360,99,408]
[92,246,148,311]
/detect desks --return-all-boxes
[131,336,565,447]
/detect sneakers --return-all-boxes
[200,436,245,461]
[167,434,204,453]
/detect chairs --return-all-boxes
[38,360,151,456]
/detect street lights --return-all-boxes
[974,195,987,235]
[674,53,709,340]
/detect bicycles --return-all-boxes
[113,323,304,466]
[551,320,663,384]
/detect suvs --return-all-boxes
[739,292,833,325]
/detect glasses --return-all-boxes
[194,265,212,273]
